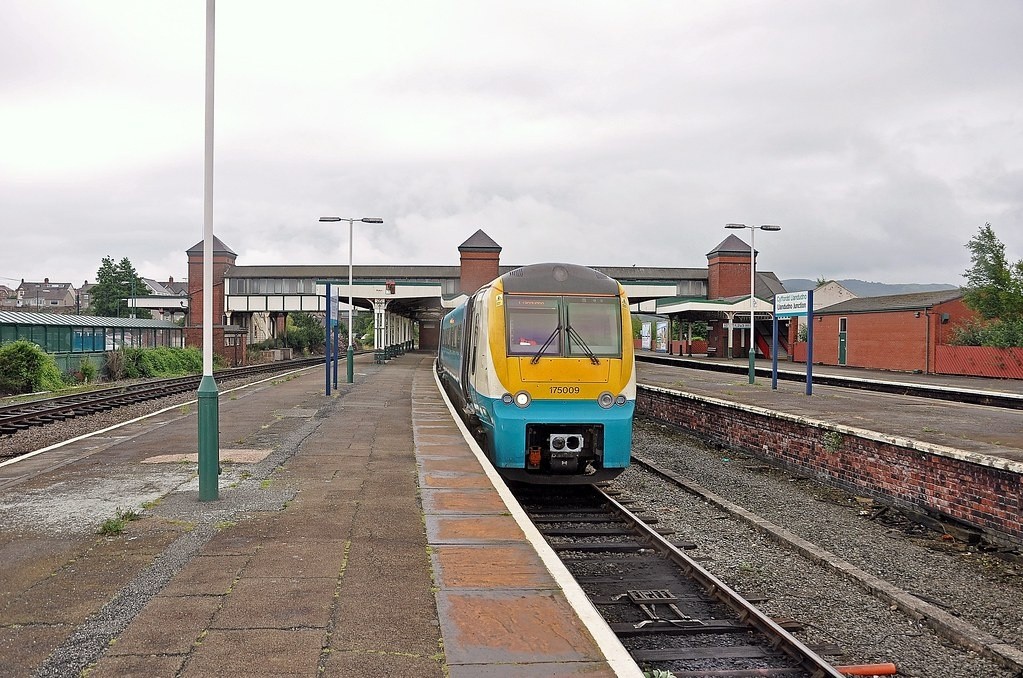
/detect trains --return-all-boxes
[437,262,638,487]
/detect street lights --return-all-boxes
[725,223,781,384]
[36,290,51,314]
[317,216,384,384]
[114,267,138,319]
